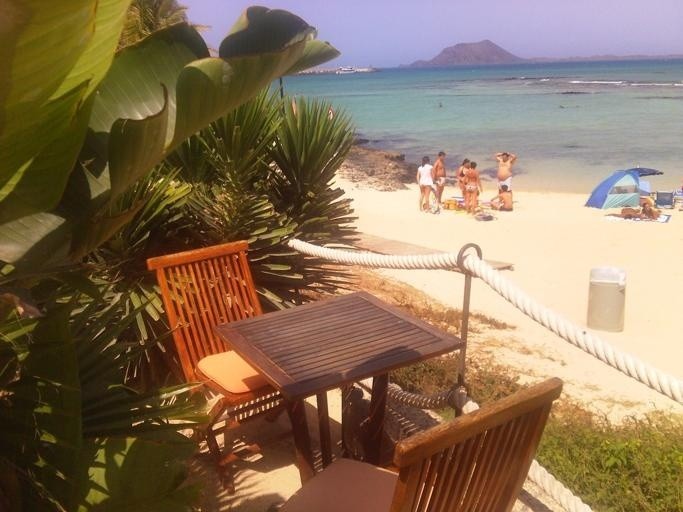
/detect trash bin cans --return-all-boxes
[587,267,628,332]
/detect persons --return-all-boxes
[433,150,446,205]
[606,205,641,220]
[417,156,436,213]
[458,158,470,199]
[462,162,483,216]
[489,184,513,211]
[494,152,516,199]
[642,201,661,220]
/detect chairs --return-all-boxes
[147,239,312,497]
[274,375,564,511]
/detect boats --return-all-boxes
[336,66,376,75]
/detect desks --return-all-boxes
[213,290,465,488]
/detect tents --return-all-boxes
[582,166,664,210]
